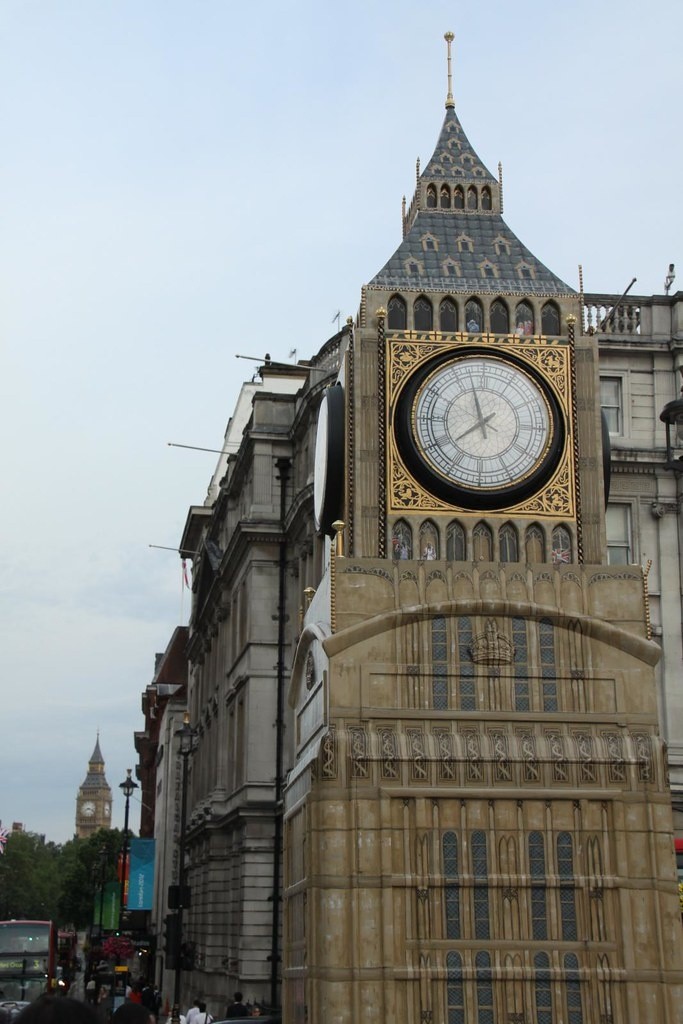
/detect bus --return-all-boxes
[1,919,78,1024]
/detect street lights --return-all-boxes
[110,769,140,990]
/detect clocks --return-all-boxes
[80,801,96,817]
[411,358,554,496]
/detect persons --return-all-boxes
[192,1002,214,1024]
[166,1003,186,1024]
[55,955,163,1021]
[226,992,248,1019]
[108,1002,157,1024]
[10,993,100,1024]
[186,1000,203,1024]
[252,1007,261,1017]
